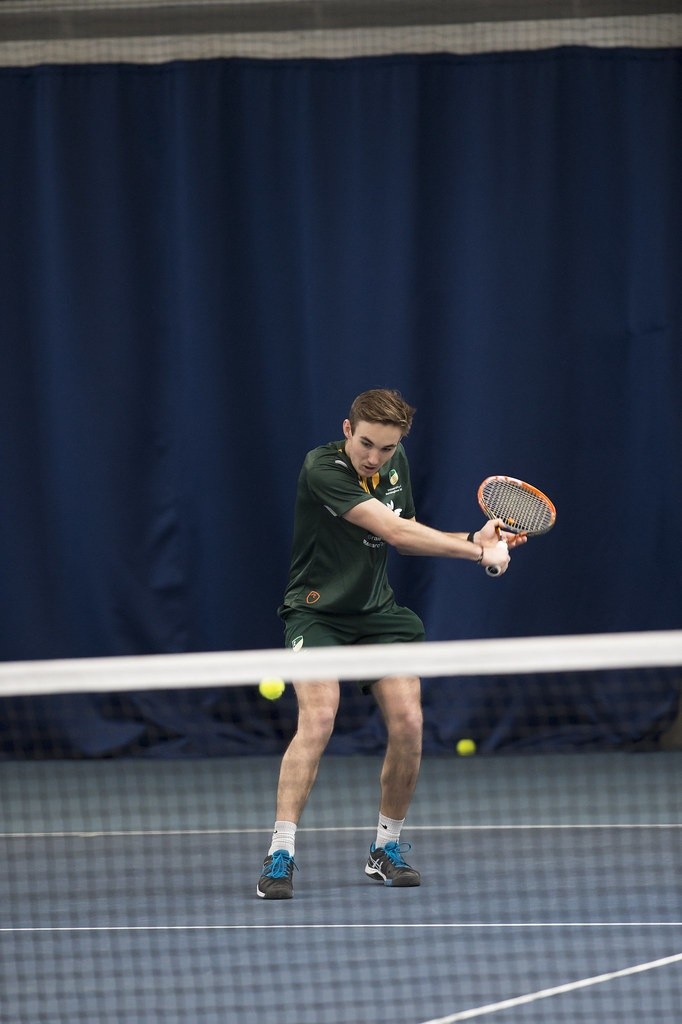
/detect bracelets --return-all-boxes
[467,531,477,543]
[476,545,483,565]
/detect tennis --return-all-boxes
[456,738,477,756]
[259,679,285,700]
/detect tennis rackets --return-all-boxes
[475,474,559,578]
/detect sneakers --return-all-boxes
[364,841,421,887]
[256,850,299,899]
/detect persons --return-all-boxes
[255,391,527,900]
